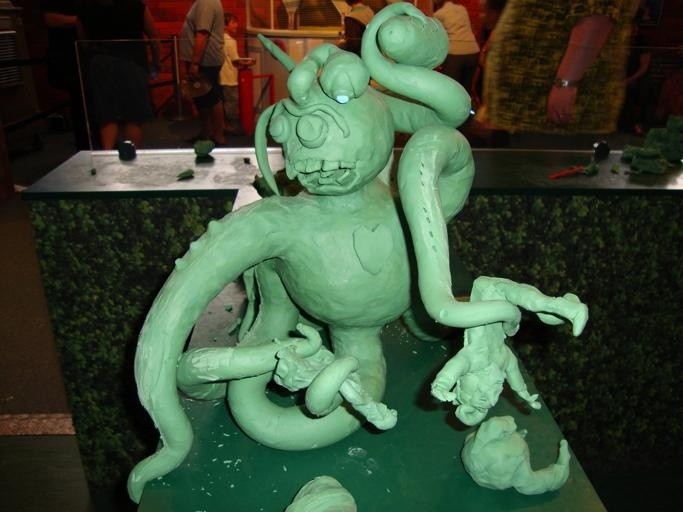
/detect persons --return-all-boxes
[429,0,479,91]
[219,11,257,136]
[178,1,229,147]
[482,1,642,150]
[616,18,650,136]
[341,0,376,54]
[75,1,162,149]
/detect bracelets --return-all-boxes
[552,75,585,89]
